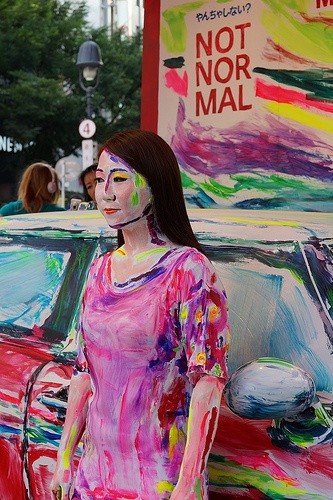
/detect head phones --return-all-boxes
[47,165,56,193]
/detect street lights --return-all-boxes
[76,41,105,140]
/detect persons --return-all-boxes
[51,130,231,500]
[0,163,66,217]
[81,163,98,209]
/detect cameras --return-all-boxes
[71,199,82,211]
